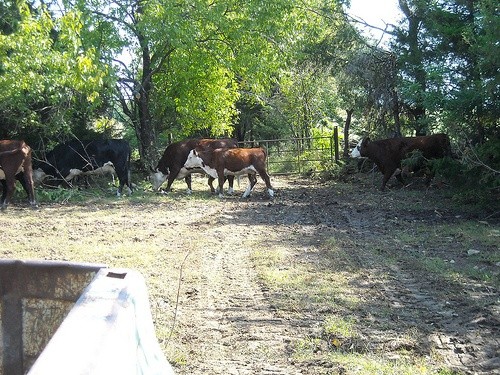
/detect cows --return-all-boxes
[148,137,239,194]
[184,147,274,198]
[350,133,450,191]
[33,139,132,197]
[0,139,36,209]
